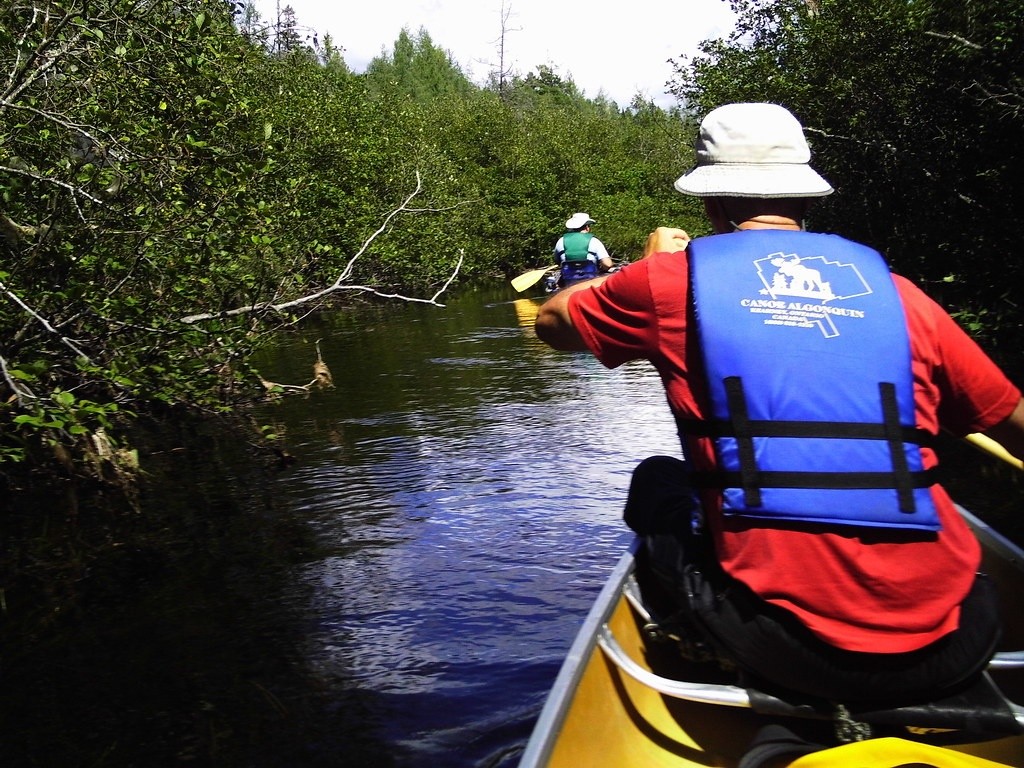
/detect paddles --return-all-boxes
[511,262,560,293]
[959,432,1024,471]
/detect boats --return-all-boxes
[544,260,629,292]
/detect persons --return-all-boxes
[551,211,614,272]
[533,101,1024,713]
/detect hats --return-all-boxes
[674,100,835,197]
[566,212,597,229]
